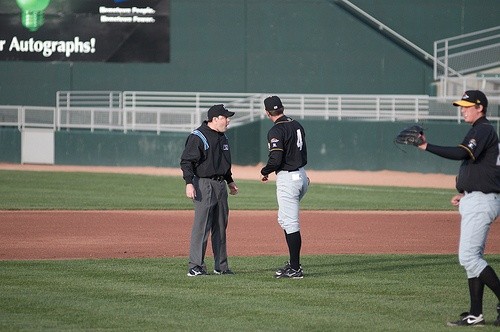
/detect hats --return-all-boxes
[264,95,283,110]
[452,89,489,110]
[207,103,235,119]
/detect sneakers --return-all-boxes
[495,304,500,327]
[271,262,304,280]
[186,265,210,276]
[447,312,487,328]
[213,268,233,275]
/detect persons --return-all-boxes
[394,90,500,326]
[180,105,239,276]
[261,96,309,279]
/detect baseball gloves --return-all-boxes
[395,126,424,146]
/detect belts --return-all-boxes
[288,168,299,172]
[210,176,225,182]
